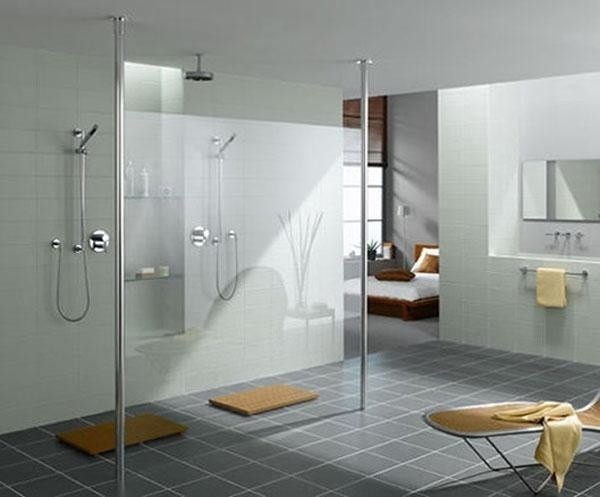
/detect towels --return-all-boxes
[492,400,583,494]
[536,268,569,309]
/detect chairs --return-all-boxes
[423,390,600,496]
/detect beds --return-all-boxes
[343,244,440,321]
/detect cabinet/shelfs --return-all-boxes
[124,193,182,284]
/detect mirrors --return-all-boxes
[520,157,600,222]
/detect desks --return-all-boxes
[286,310,335,345]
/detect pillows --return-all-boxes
[417,254,439,273]
[374,268,415,282]
[410,247,439,273]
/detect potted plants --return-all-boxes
[367,239,380,260]
